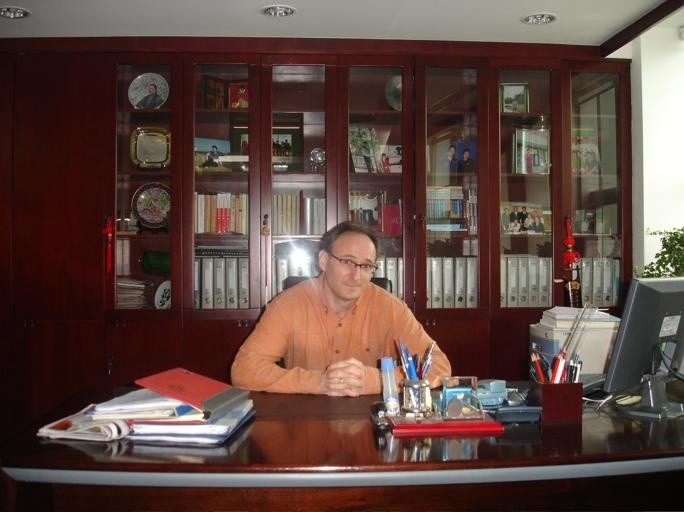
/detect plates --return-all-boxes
[126,72,168,110]
[129,181,171,229]
[127,125,171,167]
[384,75,415,111]
[153,280,171,310]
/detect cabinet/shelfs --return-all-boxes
[0,35,632,434]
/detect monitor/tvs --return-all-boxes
[603,276,684,418]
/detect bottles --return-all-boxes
[382,432,399,464]
[378,356,398,401]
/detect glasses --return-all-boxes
[327,254,379,274]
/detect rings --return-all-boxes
[338,378,344,383]
[229,220,452,397]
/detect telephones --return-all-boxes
[476,380,508,409]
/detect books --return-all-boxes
[115,278,153,309]
[195,192,249,235]
[35,366,259,458]
[272,191,327,234]
[426,187,478,233]
[349,190,403,234]
[538,302,621,329]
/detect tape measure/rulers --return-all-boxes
[563,303,599,359]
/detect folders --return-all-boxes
[579,258,620,307]
[194,257,249,309]
[501,254,553,307]
[273,255,316,299]
[426,257,480,308]
[372,257,404,299]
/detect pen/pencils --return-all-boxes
[528,343,582,384]
[394,338,436,408]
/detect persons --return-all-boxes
[381,151,391,173]
[441,145,458,171]
[136,84,163,108]
[199,145,222,168]
[272,139,291,157]
[240,140,249,155]
[524,147,533,173]
[502,206,544,233]
[570,134,598,176]
[360,141,374,173]
[458,148,477,173]
[510,98,517,112]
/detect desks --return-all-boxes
[0,388,683,511]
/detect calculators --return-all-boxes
[496,407,543,424]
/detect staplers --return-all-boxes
[370,402,391,432]
